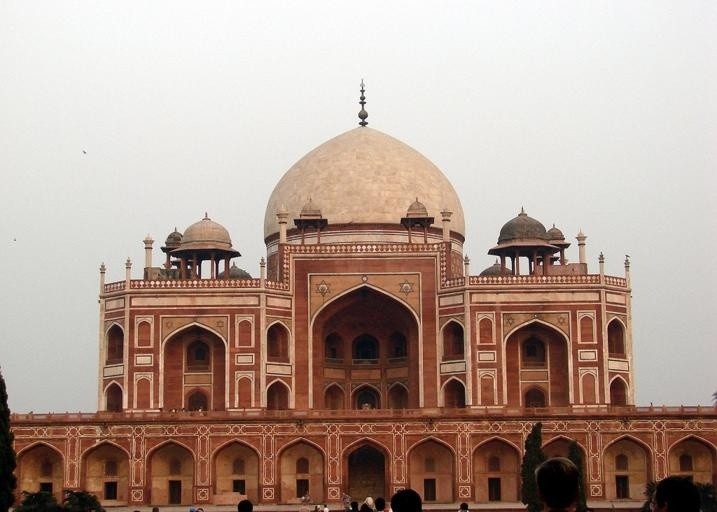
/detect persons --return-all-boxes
[313,488,422,512]
[456,502,469,511]
[237,499,253,512]
[534,455,581,511]
[650,474,702,511]
